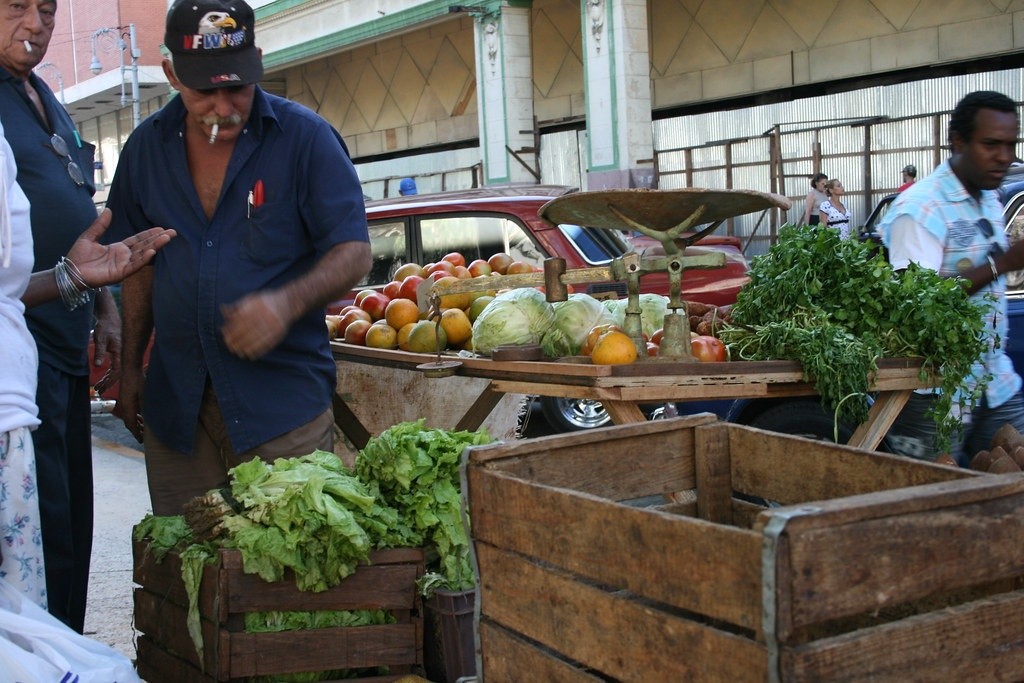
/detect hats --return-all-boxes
[164,0,264,89]
[899,165,917,176]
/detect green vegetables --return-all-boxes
[135,418,498,683]
[710,221,1002,452]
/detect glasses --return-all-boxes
[976,217,1003,259]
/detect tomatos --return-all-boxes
[324,252,573,345]
[581,324,728,363]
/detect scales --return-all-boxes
[418,190,790,377]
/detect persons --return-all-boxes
[397,177,417,196]
[818,179,852,241]
[96,1,373,519]
[0,0,121,635]
[805,172,828,235]
[0,120,178,613]
[897,165,917,193]
[876,90,1023,468]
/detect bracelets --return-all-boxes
[52,255,100,312]
[986,255,998,280]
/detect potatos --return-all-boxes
[683,301,735,335]
[934,423,1024,474]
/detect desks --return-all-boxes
[327,338,938,504]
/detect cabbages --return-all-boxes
[603,299,622,312]
[471,288,554,354]
[539,292,617,358]
[611,293,685,341]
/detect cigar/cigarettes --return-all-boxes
[208,124,219,144]
[23,39,33,53]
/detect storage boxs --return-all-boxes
[467,414,1024,683]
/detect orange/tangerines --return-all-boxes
[364,276,502,354]
[591,330,637,365]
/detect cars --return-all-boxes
[89,186,755,434]
[663,161,1023,506]
